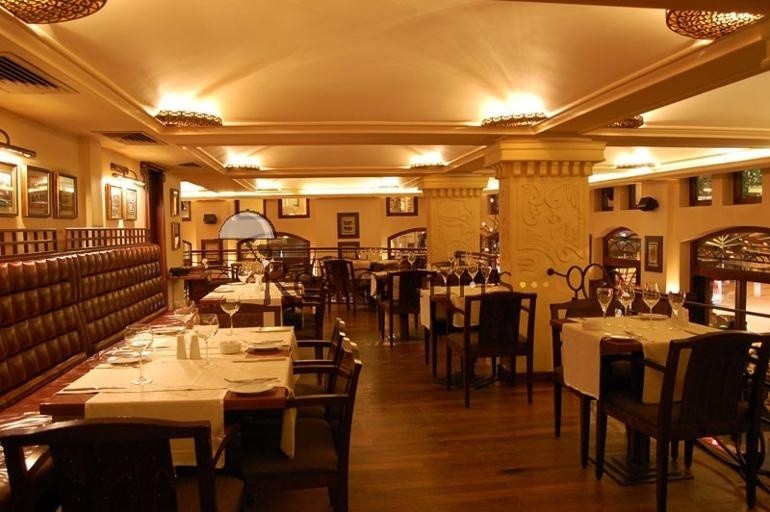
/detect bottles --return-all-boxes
[176,335,186,359]
[189,335,201,360]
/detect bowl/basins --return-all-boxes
[219,340,241,354]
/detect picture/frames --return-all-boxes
[386,196,418,216]
[0,162,78,219]
[170,188,191,250]
[337,211,360,238]
[106,184,137,220]
[645,235,663,274]
[278,198,309,219]
[338,241,359,259]
[201,238,224,265]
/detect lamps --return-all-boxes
[664,8,764,39]
[0,129,37,158]
[0,0,107,24]
[480,113,546,128]
[637,197,659,211]
[155,110,221,126]
[111,162,146,185]
[606,116,644,128]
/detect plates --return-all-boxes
[214,287,234,292]
[608,334,635,341]
[154,327,185,334]
[641,314,669,320]
[107,357,150,364]
[248,345,277,351]
[227,282,243,285]
[229,382,274,395]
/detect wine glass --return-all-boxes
[125,324,153,384]
[669,290,686,329]
[618,285,636,327]
[237,263,265,295]
[193,314,220,370]
[596,287,613,319]
[439,262,492,289]
[395,250,476,271]
[641,282,661,324]
[173,299,195,335]
[220,294,241,336]
[356,247,383,263]
